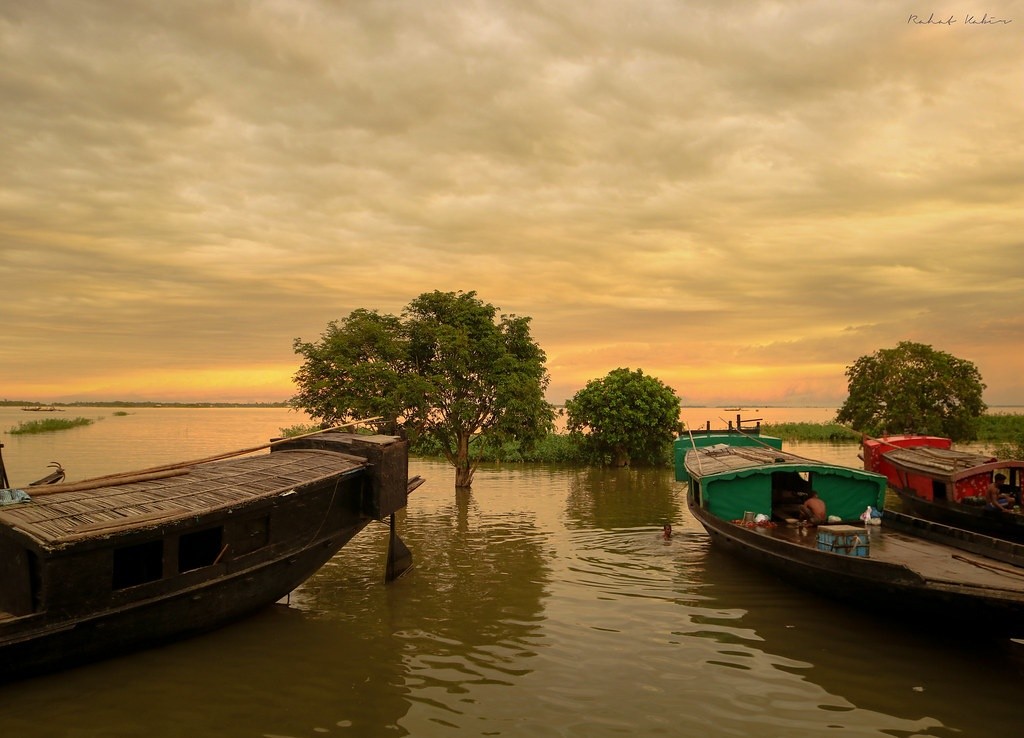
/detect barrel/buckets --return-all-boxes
[745,512,754,522]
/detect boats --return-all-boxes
[857,428,1024,538]
[672,417,1024,618]
[0,409,427,677]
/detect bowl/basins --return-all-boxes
[786,519,799,524]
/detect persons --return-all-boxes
[799,491,826,527]
[986,473,1016,513]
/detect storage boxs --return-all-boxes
[818,526,868,558]
